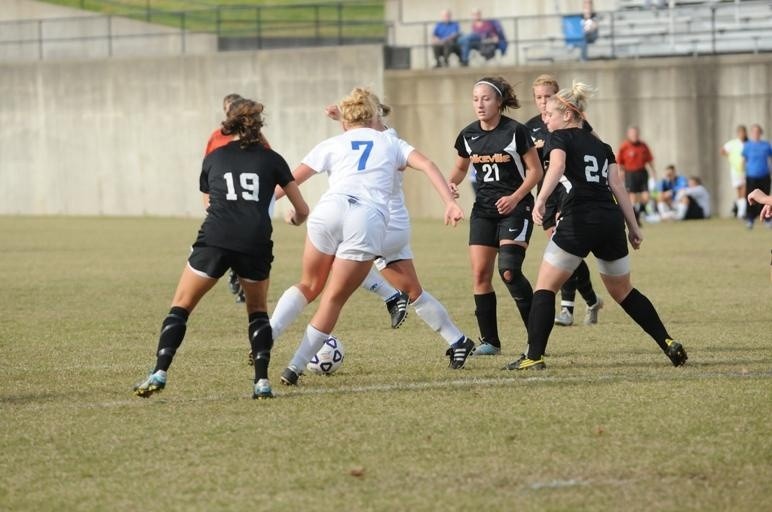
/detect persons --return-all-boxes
[447,78,542,356]
[566,1,599,60]
[248,89,464,387]
[133,100,310,399]
[500,81,688,370]
[616,126,656,227]
[455,9,500,67]
[742,125,771,229]
[206,95,275,303]
[325,97,476,371]
[647,165,712,221]
[523,73,604,327]
[431,10,462,67]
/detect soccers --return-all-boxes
[306,335,344,375]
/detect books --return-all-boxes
[721,126,746,219]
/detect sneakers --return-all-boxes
[762,218,770,228]
[583,296,604,328]
[132,369,167,398]
[235,294,246,303]
[250,378,276,402]
[665,341,688,367]
[247,350,254,367]
[278,368,299,387]
[467,338,502,355]
[554,306,574,326]
[446,336,475,370]
[228,269,241,294]
[384,289,410,329]
[744,221,756,232]
[500,355,546,372]
